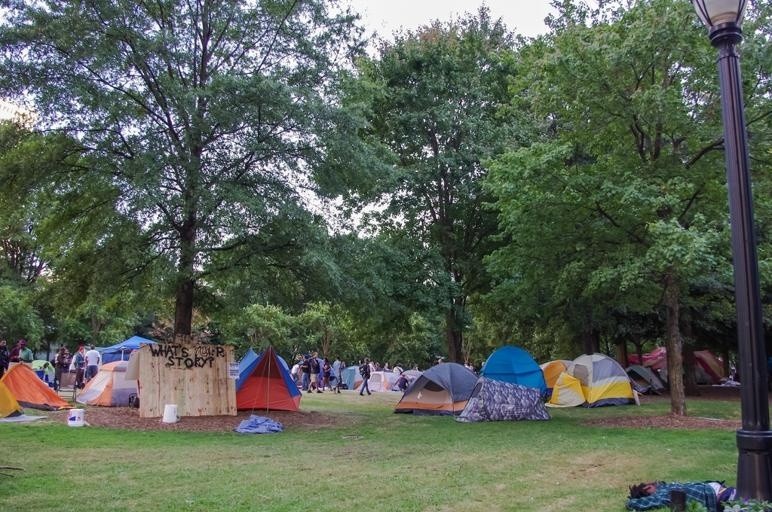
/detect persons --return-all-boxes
[292,351,408,396]
[1,339,69,391]
[625,476,738,512]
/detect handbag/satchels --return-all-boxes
[324,367,331,372]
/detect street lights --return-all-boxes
[690,0,772,507]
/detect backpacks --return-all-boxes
[359,365,367,375]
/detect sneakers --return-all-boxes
[307,386,340,393]
[360,392,371,395]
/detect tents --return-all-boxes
[0,364,72,419]
[235,345,301,411]
[77,336,157,408]
[340,346,724,422]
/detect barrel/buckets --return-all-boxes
[66,408,85,427]
[162,404,181,423]
